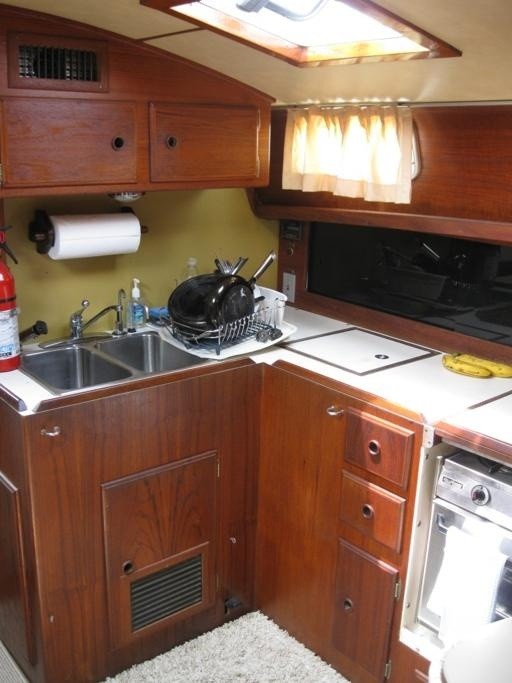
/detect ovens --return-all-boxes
[416,453,510,639]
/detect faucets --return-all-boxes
[113,289,127,336]
[70,299,122,337]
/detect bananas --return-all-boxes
[442,353,512,378]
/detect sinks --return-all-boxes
[94,329,218,376]
[16,343,134,398]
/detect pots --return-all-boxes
[168,250,278,326]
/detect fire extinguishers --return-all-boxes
[0,225,22,373]
